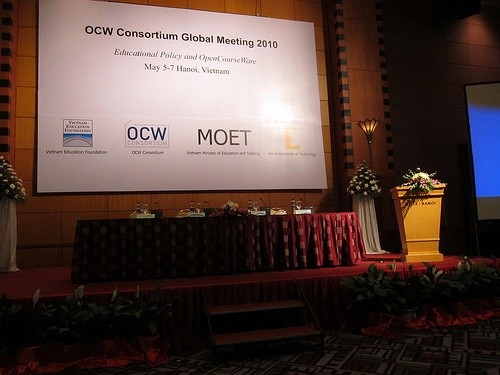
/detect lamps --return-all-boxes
[358,117,380,145]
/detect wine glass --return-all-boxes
[253,202,259,211]
[195,203,201,213]
[142,204,148,214]
[296,201,301,210]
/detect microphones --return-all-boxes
[204,201,211,208]
[300,198,306,206]
[259,199,266,206]
[154,202,159,208]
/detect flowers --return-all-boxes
[342,255,500,317]
[0,155,27,206]
[0,283,179,342]
[403,166,442,193]
[347,169,382,200]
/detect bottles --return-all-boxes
[189,200,195,213]
[248,200,253,211]
[136,202,141,214]
[291,199,295,211]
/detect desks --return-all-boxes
[72,210,367,285]
